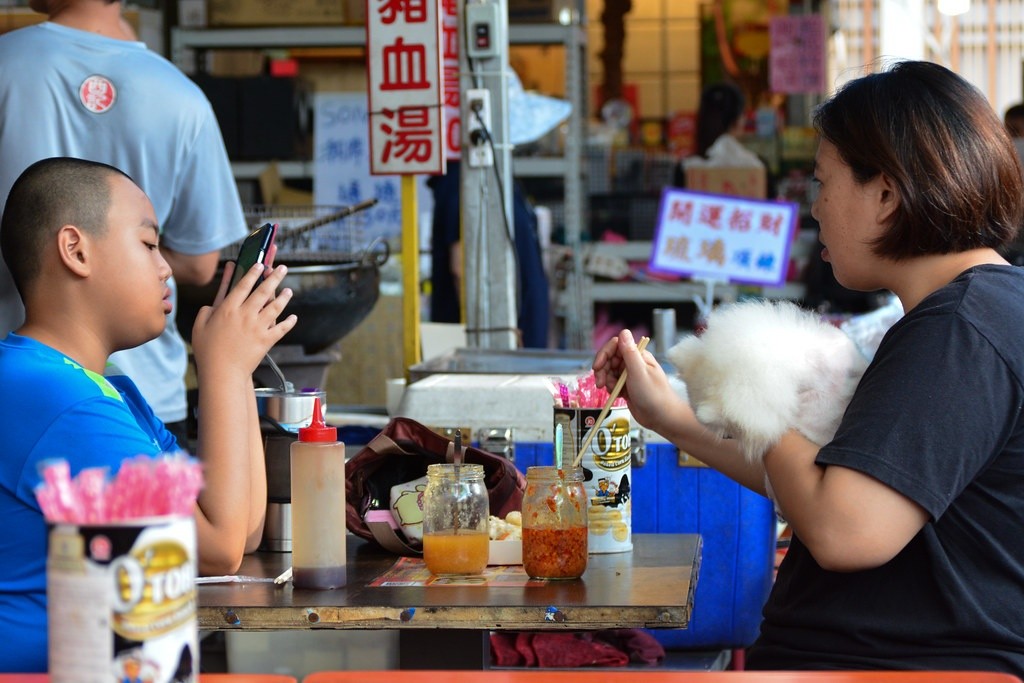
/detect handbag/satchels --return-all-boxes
[344,416,525,564]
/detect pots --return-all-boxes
[176,237,392,355]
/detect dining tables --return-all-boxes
[192,533,703,671]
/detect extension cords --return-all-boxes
[468,87,495,169]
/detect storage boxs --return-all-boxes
[394,373,776,648]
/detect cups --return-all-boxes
[553,404,633,554]
[46,513,200,683]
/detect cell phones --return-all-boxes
[222,222,273,298]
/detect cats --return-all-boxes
[661,296,865,525]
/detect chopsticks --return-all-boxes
[573,336,650,467]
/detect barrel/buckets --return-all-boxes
[254,383,326,554]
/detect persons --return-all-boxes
[428,55,576,349]
[670,81,785,204]
[1005,102,1024,163]
[590,59,1024,683]
[0,0,299,683]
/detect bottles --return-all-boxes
[522,465,589,580]
[423,464,490,577]
[291,398,347,590]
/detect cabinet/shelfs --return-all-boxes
[171,0,593,351]
